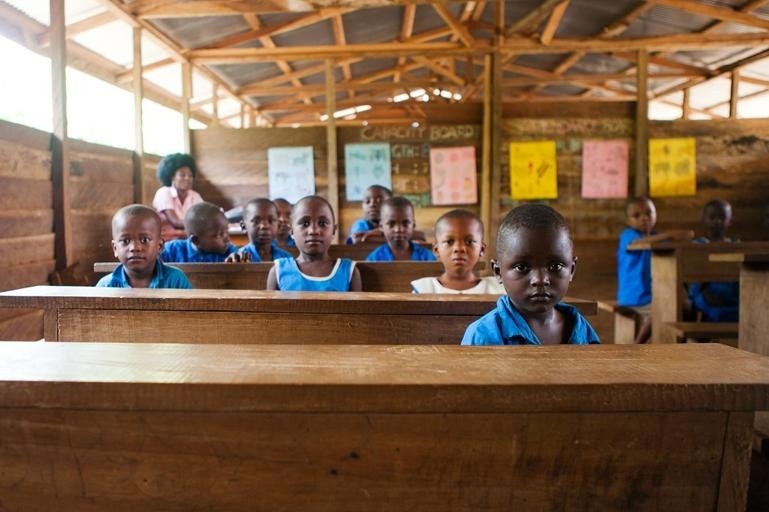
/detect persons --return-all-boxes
[408,209,509,297]
[615,198,695,344]
[692,199,746,319]
[152,155,205,239]
[459,205,606,345]
[271,200,298,245]
[95,203,193,288]
[236,198,293,260]
[264,196,364,292]
[349,186,426,244]
[364,198,438,261]
[158,202,252,264]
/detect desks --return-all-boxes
[0,236,769,511]
